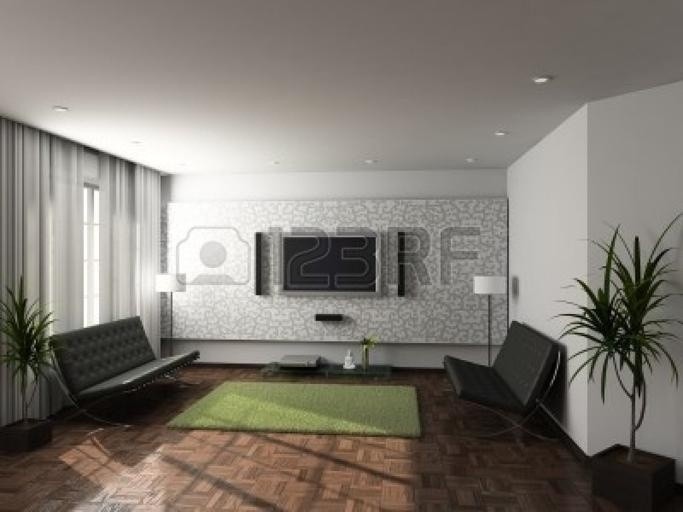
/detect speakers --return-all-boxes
[256,232,261,295]
[316,314,344,321]
[398,231,405,296]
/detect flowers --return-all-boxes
[356,335,378,351]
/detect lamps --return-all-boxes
[156,273,186,356]
[473,276,509,364]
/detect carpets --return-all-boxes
[168,380,422,440]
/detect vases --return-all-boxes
[361,348,369,369]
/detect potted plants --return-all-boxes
[553,210,682,510]
[0,276,54,452]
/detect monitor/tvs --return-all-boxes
[283,236,382,296]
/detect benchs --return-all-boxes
[443,321,562,443]
[46,315,199,429]
